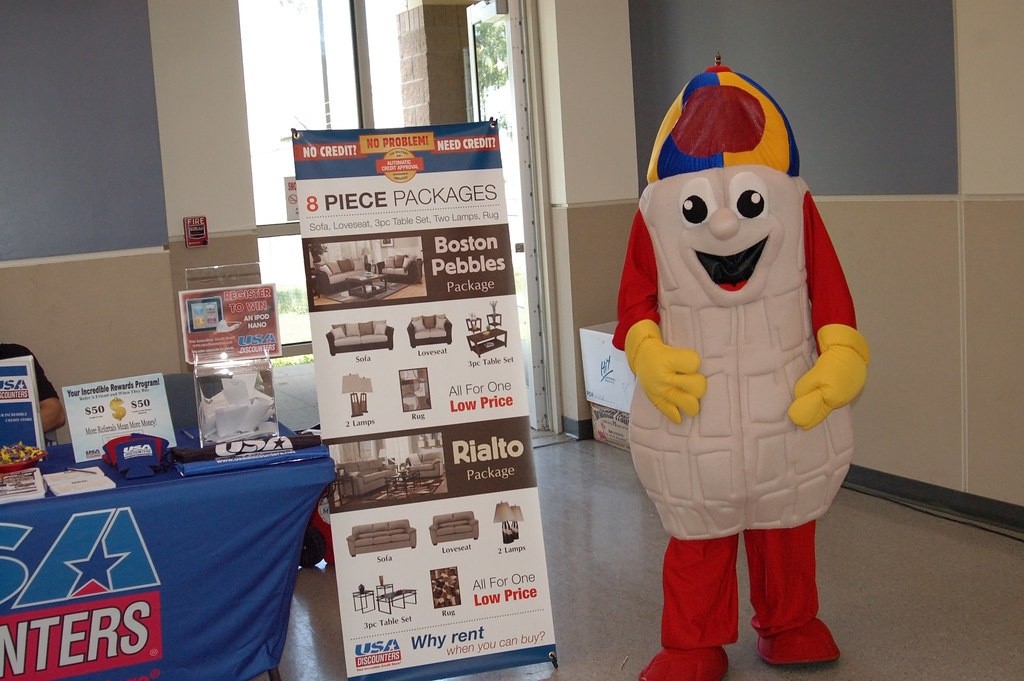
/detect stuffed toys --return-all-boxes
[609,53,874,681]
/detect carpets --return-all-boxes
[323,279,410,304]
[398,366,432,412]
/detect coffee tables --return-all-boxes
[345,273,388,297]
[385,471,420,496]
[465,328,507,360]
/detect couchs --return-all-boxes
[405,452,443,479]
[335,454,396,497]
[406,313,453,349]
[375,254,423,285]
[313,256,367,295]
[428,510,482,546]
[347,520,418,558]
[325,320,395,356]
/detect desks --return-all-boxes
[352,589,376,616]
[375,581,417,614]
[0,415,338,681]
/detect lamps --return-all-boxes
[342,372,361,418]
[508,504,524,541]
[355,376,372,415]
[378,449,388,467]
[493,502,516,546]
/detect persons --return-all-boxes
[0,341,67,438]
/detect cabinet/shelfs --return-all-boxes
[487,300,502,328]
[466,317,482,333]
